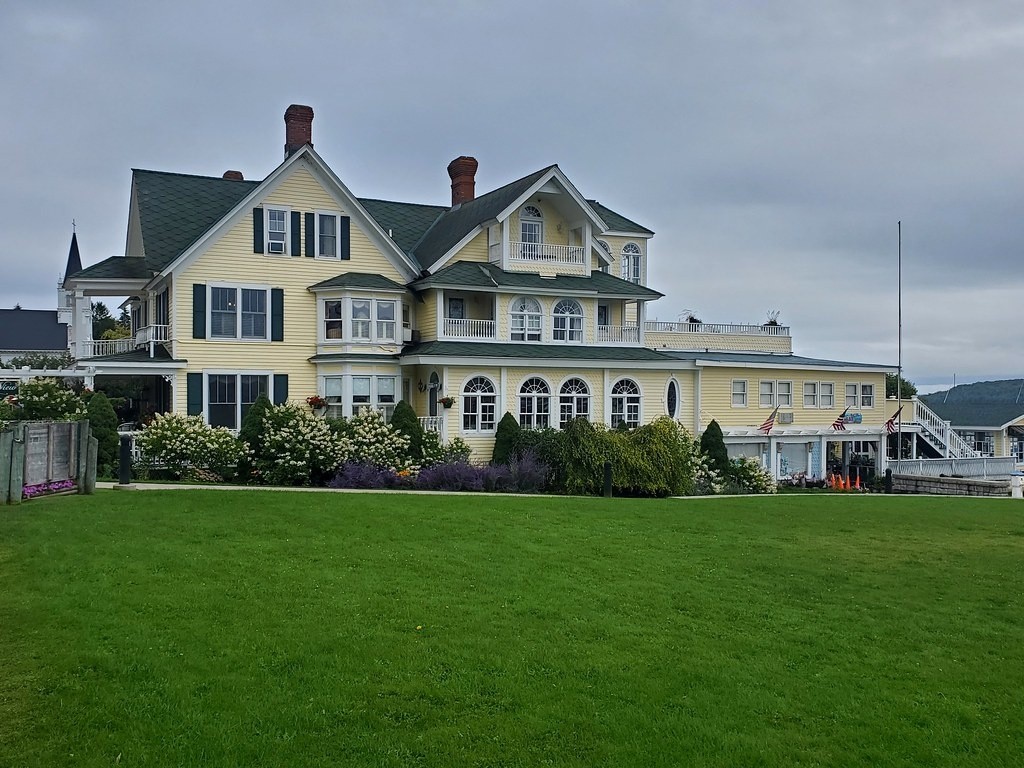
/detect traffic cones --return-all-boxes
[845,476,851,489]
[832,474,836,486]
[838,474,844,488]
[855,476,859,488]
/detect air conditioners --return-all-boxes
[779,412,793,424]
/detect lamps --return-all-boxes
[417,379,427,394]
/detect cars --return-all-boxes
[116,422,140,436]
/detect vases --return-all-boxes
[443,402,453,408]
[314,403,323,410]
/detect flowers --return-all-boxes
[306,395,331,416]
[436,395,456,403]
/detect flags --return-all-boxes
[886,407,904,434]
[756,406,780,434]
[832,408,849,430]
